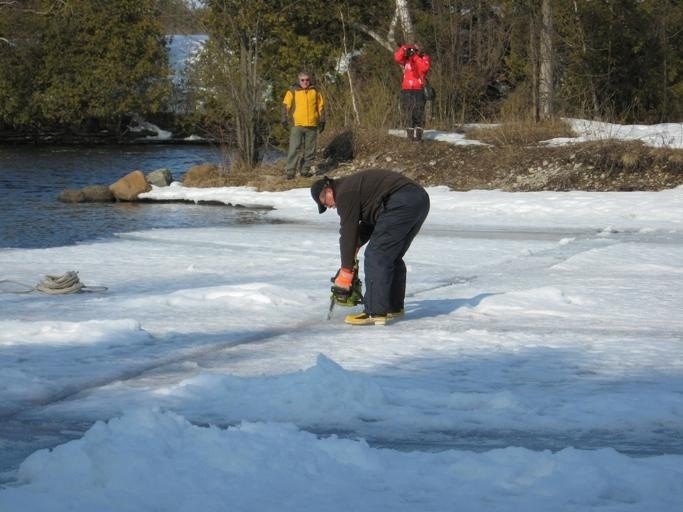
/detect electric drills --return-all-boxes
[322,260,362,321]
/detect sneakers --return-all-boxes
[287,173,312,180]
[345,310,404,325]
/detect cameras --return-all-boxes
[407,48,414,56]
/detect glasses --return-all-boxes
[300,79,310,81]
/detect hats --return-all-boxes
[310,179,329,213]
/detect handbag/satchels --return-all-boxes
[422,84,436,100]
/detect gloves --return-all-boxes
[281,121,290,132]
[336,268,355,290]
[319,124,325,132]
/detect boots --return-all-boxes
[406,128,423,143]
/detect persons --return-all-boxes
[280,71,329,181]
[393,37,432,142]
[310,168,432,327]
